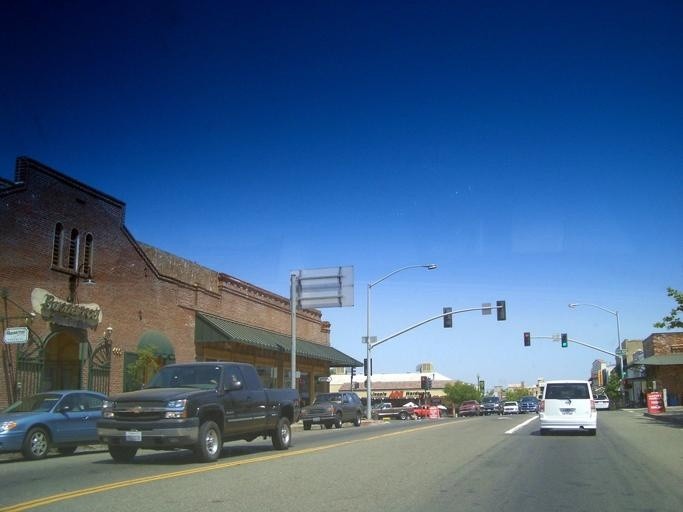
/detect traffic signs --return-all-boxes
[616,349,628,356]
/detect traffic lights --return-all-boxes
[522,332,531,347]
[418,375,432,391]
[560,333,568,348]
[350,366,357,378]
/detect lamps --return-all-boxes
[103,323,112,342]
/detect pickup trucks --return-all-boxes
[411,406,430,418]
[96,360,302,463]
[371,402,414,421]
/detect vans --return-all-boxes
[538,380,596,437]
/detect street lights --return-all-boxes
[568,302,625,408]
[366,262,438,423]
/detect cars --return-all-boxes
[0,389,112,460]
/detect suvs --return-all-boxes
[300,391,365,430]
[515,396,538,414]
[478,396,504,416]
[502,401,519,415]
[457,400,480,418]
[592,388,609,411]
